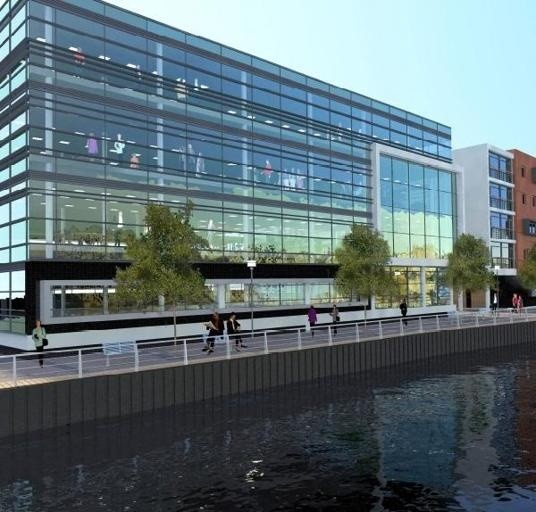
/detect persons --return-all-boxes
[308,305,317,337]
[511,293,523,312]
[400,299,407,326]
[330,304,339,334]
[202,312,225,354]
[87,133,311,192]
[32,320,46,369]
[492,293,498,310]
[227,312,248,352]
